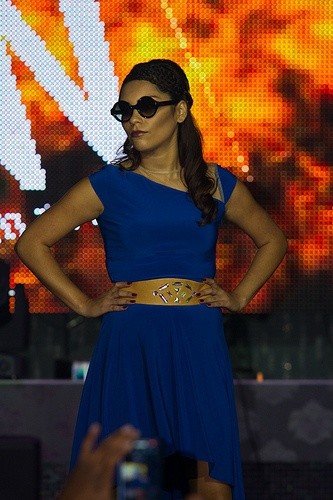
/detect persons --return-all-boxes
[13,59,289,499]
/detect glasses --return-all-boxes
[110,96,176,122]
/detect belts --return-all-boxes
[118,278,214,305]
